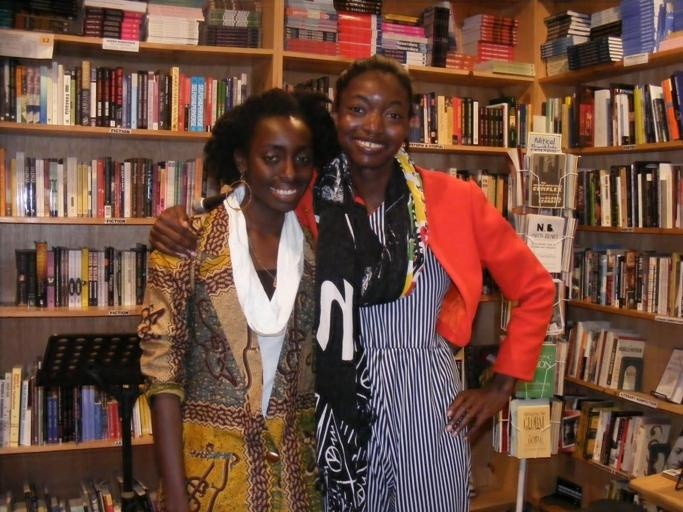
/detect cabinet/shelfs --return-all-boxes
[1,0,528,512]
[525,0,683,512]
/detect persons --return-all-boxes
[145,56,559,512]
[134,85,345,512]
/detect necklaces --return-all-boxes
[242,224,278,292]
